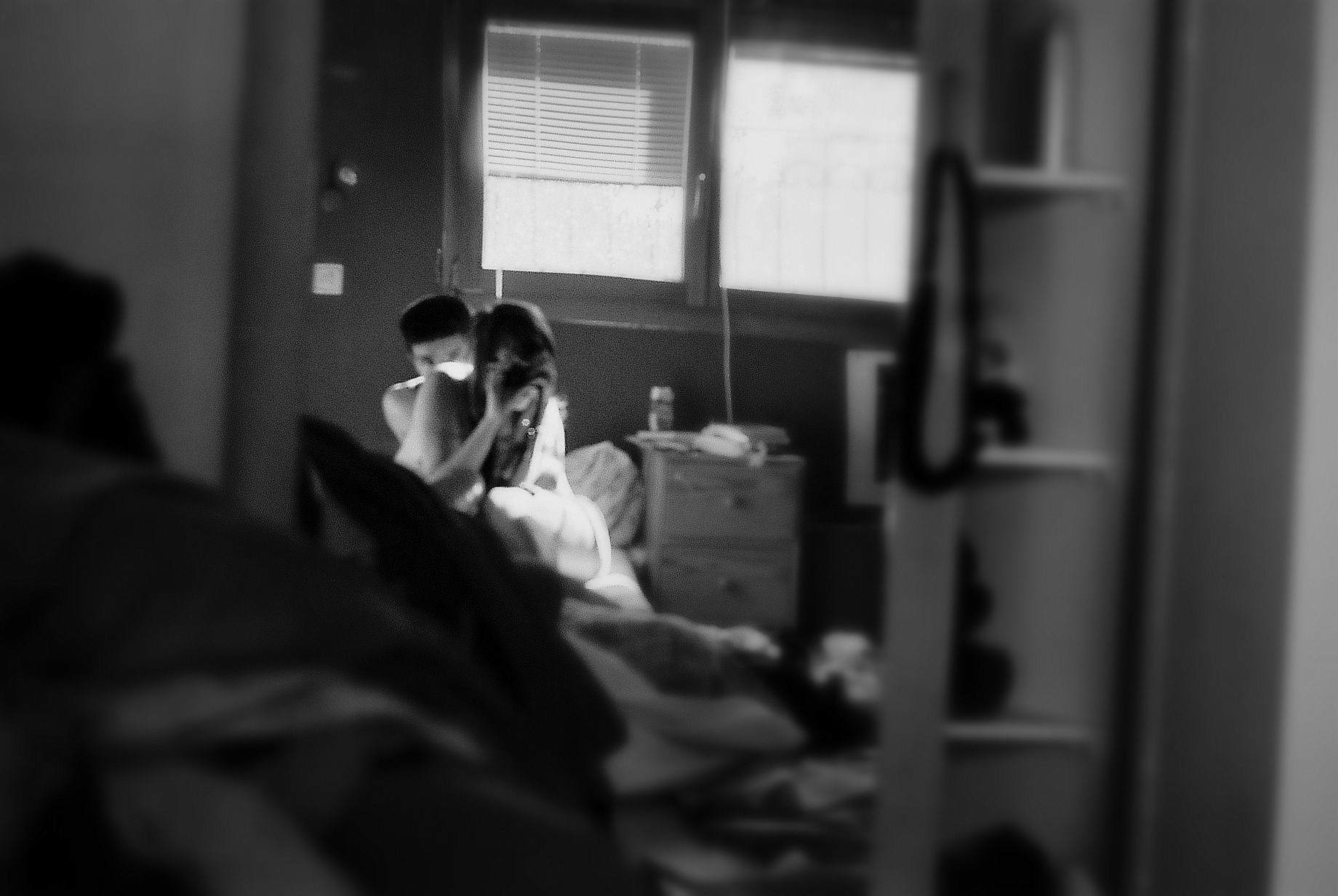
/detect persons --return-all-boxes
[392,300,558,524]
[382,296,475,445]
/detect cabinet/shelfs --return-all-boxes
[876,0,1149,894]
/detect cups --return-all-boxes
[648,386,675,431]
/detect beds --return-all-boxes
[562,440,655,610]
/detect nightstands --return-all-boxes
[625,435,801,634]
